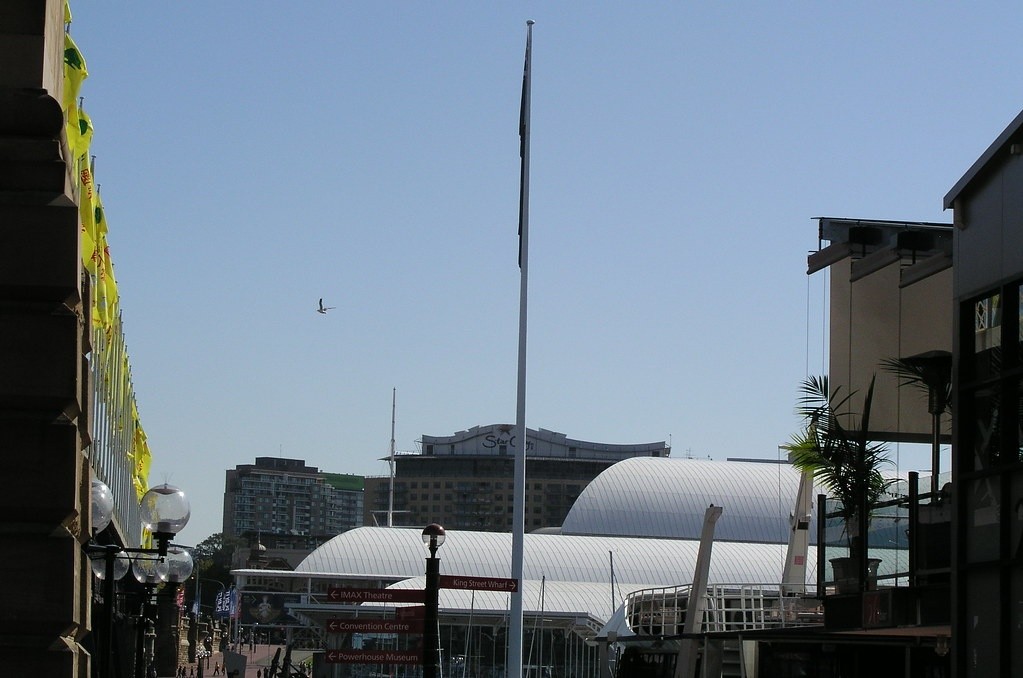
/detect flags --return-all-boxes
[63,1,99,277]
[90,197,154,550]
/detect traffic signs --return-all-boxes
[327,587,425,603]
[438,574,519,593]
[324,648,422,664]
[326,617,424,634]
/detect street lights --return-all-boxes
[239,626,243,656]
[249,622,260,666]
[421,523,446,678]
[89,478,192,678]
[92,547,196,678]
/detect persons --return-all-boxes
[213,662,219,675]
[300,662,306,673]
[307,662,311,676]
[257,669,261,678]
[178,666,182,678]
[263,667,269,678]
[195,665,198,678]
[182,667,187,678]
[189,666,194,678]
[221,662,225,676]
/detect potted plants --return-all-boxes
[777,372,902,595]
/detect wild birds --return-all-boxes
[316,297,336,315]
